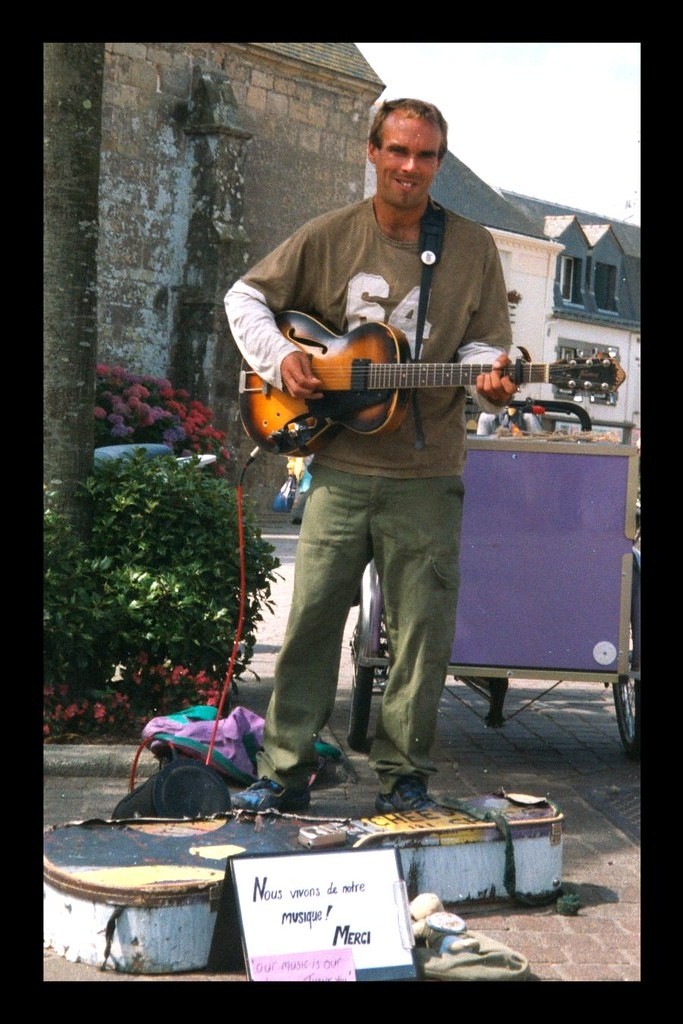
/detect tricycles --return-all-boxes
[338,394,642,763]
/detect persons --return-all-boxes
[223,100,517,817]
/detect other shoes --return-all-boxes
[291,519,301,525]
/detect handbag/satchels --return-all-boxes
[141,704,341,790]
[273,474,296,513]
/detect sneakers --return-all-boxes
[375,777,440,813]
[229,777,311,812]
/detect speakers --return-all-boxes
[109,759,231,820]
